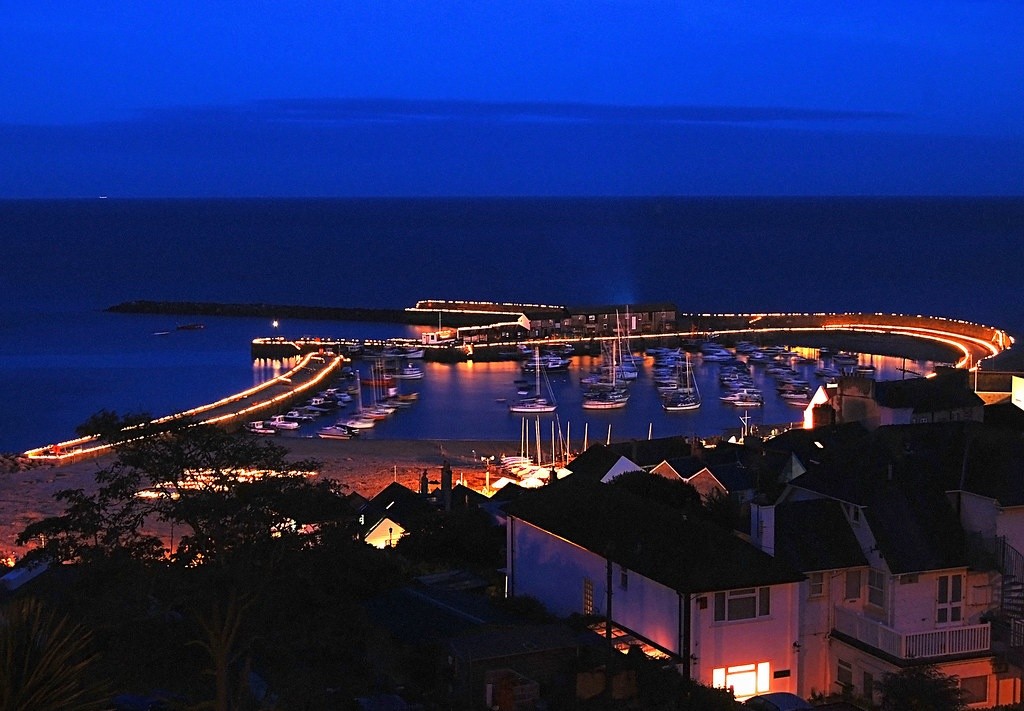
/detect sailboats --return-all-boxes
[498,414,590,487]
[507,344,573,413]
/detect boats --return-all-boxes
[243,419,276,435]
[580,306,875,411]
[294,359,425,440]
[285,411,315,423]
[265,414,302,431]
